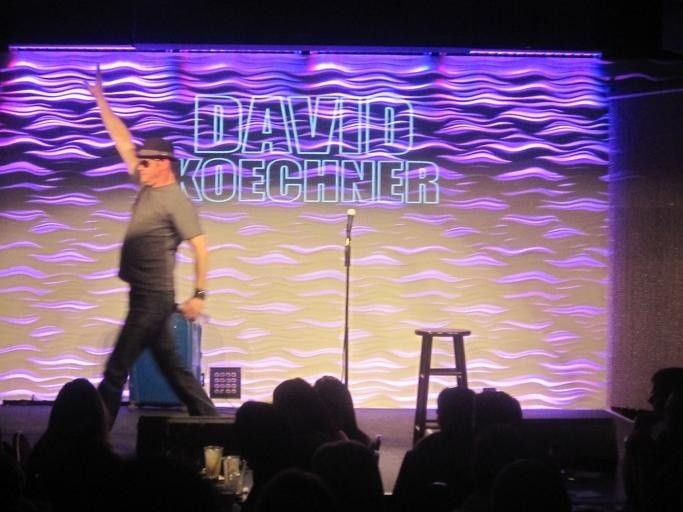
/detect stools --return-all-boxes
[413,327,472,448]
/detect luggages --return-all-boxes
[129,303,202,411]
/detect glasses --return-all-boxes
[140,158,150,167]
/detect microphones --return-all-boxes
[342,208,356,235]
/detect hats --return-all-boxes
[136,137,177,160]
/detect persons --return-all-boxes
[235,375,383,512]
[24,378,132,512]
[76,55,220,420]
[621,370,683,512]
[390,388,561,512]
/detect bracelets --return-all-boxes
[193,286,207,300]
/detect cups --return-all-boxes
[202,445,242,488]
